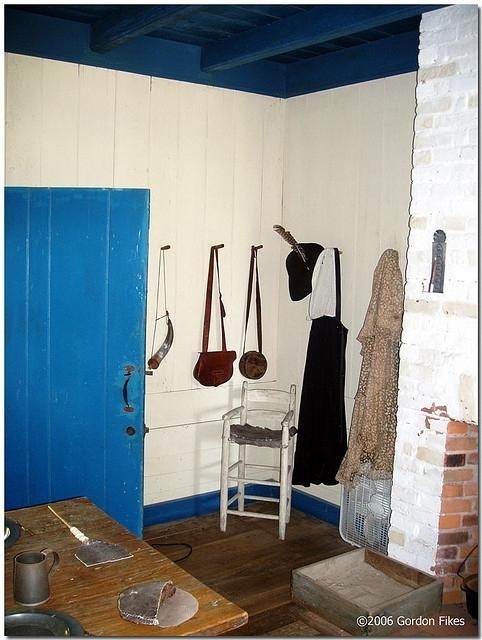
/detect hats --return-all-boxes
[285,242,325,302]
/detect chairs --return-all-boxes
[218,380,299,540]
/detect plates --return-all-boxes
[4,612,72,640]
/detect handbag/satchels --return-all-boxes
[193,349,237,387]
[238,350,268,380]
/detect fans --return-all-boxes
[338,460,393,557]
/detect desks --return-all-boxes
[4,496,249,637]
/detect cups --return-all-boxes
[13,547,61,607]
